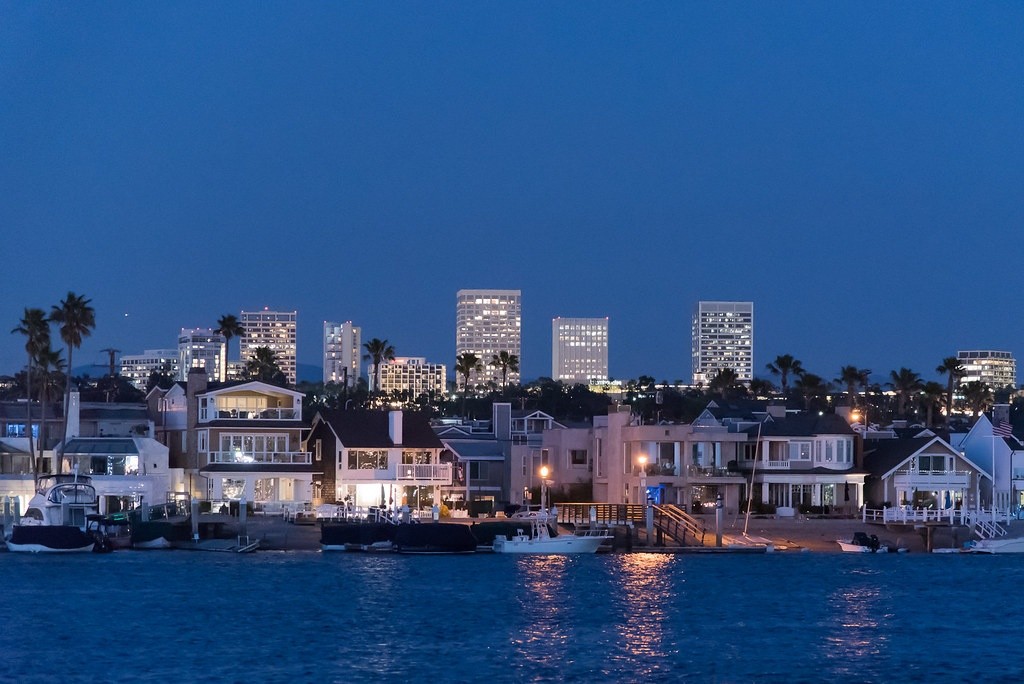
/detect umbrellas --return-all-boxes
[844,480,850,503]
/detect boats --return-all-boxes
[491,511,615,554]
[319,514,557,551]
[970,536,1024,552]
[836,531,912,553]
[3,467,101,554]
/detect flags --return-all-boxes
[992,417,1012,438]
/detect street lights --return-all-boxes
[637,454,649,504]
[539,465,549,512]
